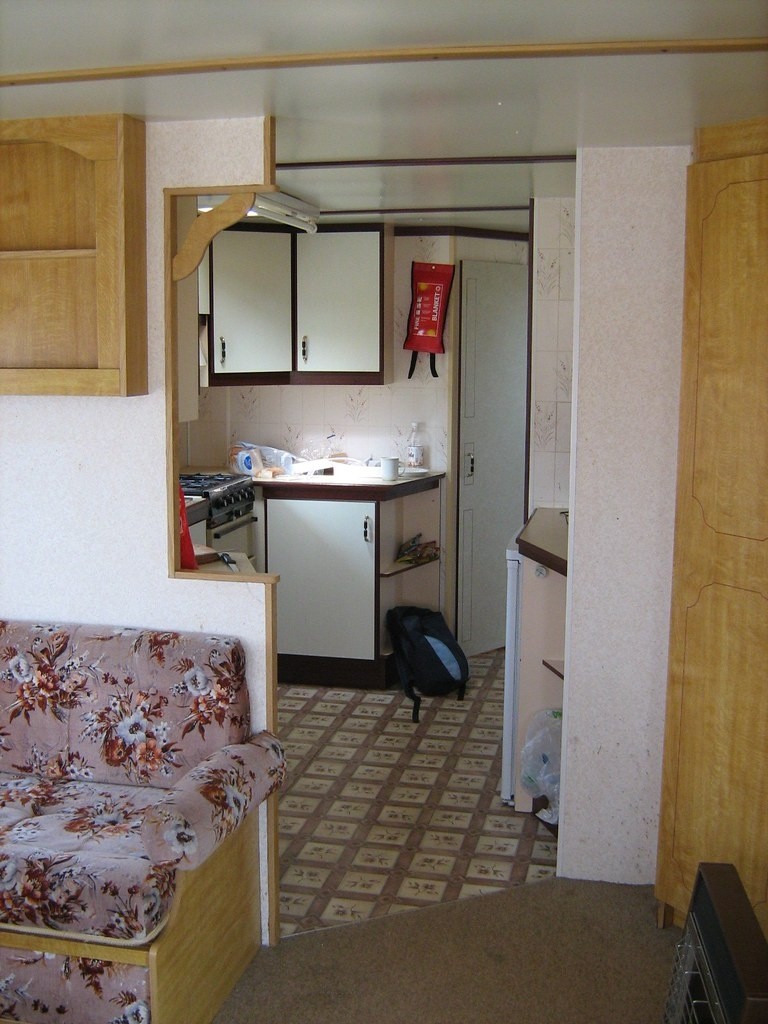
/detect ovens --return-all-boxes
[205,511,257,575]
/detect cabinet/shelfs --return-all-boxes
[253,472,446,689]
[198,222,394,388]
[188,512,254,572]
[506,117,768,928]
[0,113,146,396]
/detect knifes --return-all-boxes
[221,552,240,574]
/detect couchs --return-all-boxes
[0,620,286,1024]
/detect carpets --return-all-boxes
[209,875,668,1024]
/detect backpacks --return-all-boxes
[386,605,471,724]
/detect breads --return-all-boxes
[229,446,284,477]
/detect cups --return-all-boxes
[381,455,405,481]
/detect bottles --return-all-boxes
[403,422,423,466]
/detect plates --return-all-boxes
[396,466,429,479]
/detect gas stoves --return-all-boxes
[177,472,256,527]
